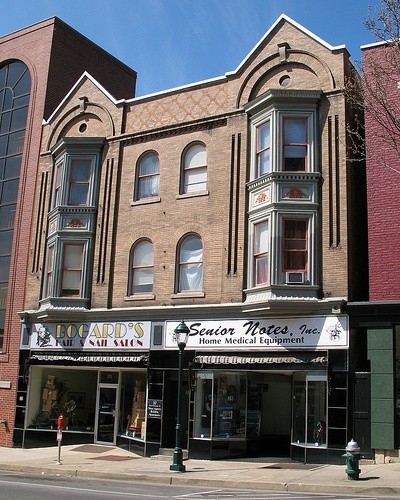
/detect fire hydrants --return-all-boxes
[342,438,364,480]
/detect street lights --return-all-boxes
[170,320,190,472]
